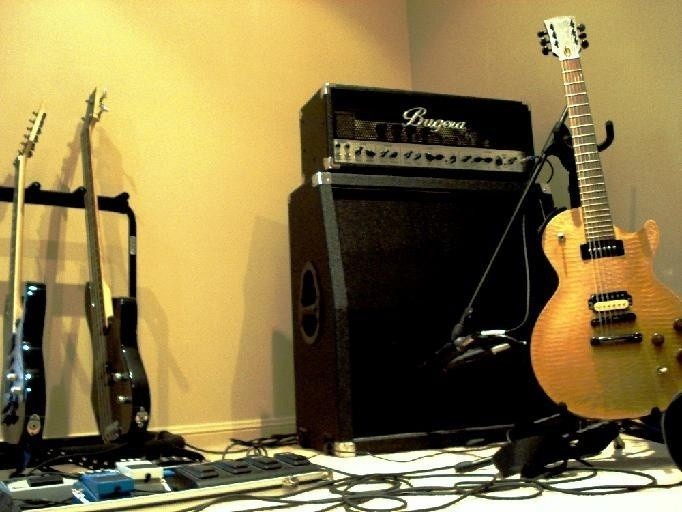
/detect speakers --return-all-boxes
[286,173,562,456]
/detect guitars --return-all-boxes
[0,108,46,449]
[528,15,682,420]
[79,88,152,446]
